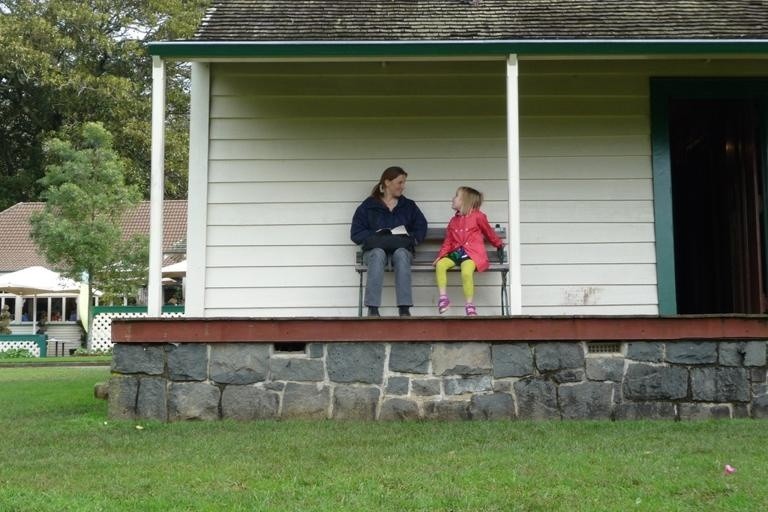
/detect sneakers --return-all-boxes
[438,296,451,313]
[398,306,412,317]
[465,303,476,315]
[367,306,379,316]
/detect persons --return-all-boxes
[40,310,48,321]
[22,310,29,321]
[51,311,62,321]
[167,294,179,305]
[350,165,428,316]
[431,186,505,316]
[70,311,76,320]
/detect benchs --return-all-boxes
[355,223,510,317]
